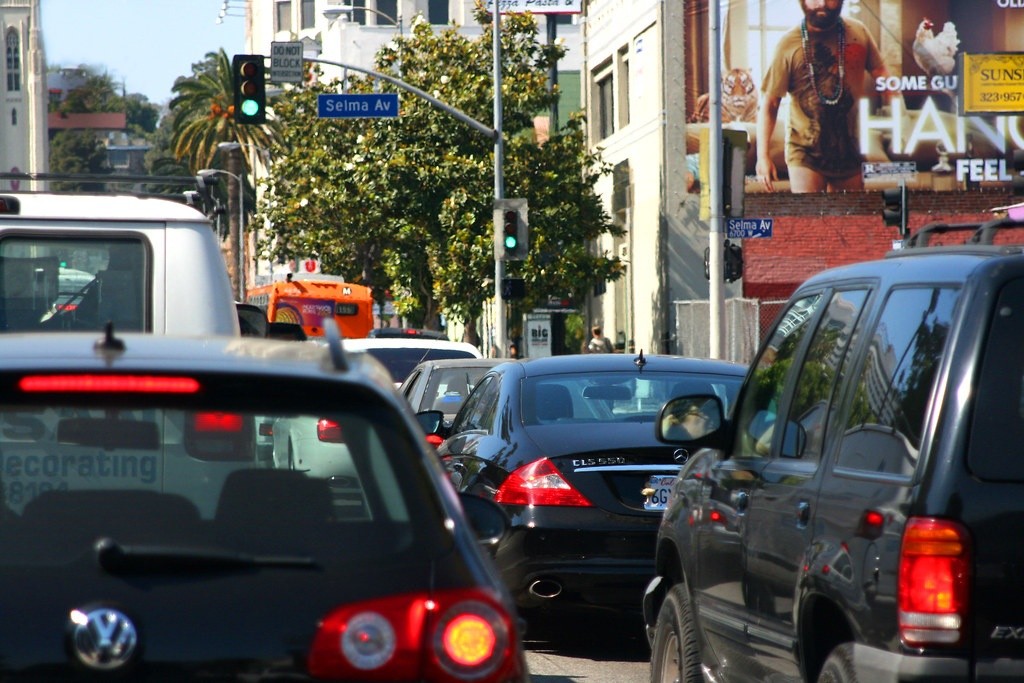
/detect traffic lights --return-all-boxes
[498,198,530,262]
[233,54,270,124]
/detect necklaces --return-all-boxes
[801,14,845,105]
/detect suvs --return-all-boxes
[642,218,1024,683]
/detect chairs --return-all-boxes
[537,385,574,422]
[673,380,716,400]
[434,378,469,414]
[78,268,140,327]
[215,467,337,551]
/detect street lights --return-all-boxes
[220,140,274,276]
[200,167,244,303]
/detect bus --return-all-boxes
[247,273,374,338]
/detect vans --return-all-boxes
[1,189,259,527]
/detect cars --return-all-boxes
[272,326,490,480]
[0,320,528,683]
[412,352,751,628]
[393,358,615,471]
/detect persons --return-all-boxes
[755,0,889,193]
[505,325,615,358]
